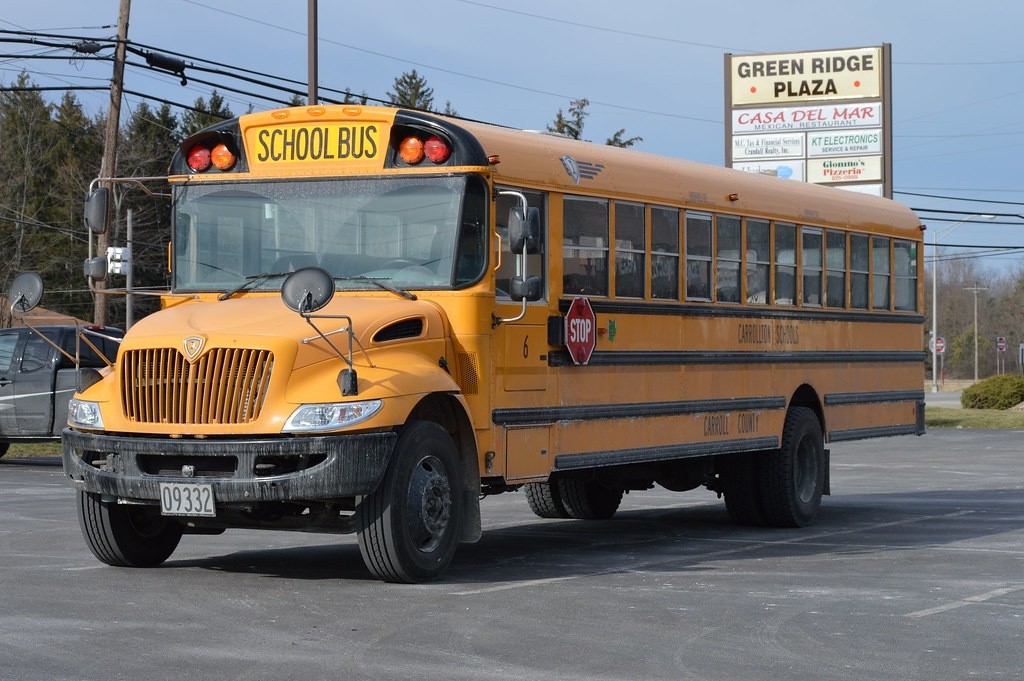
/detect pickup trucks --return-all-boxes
[0,324,125,459]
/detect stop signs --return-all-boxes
[997,337,1006,352]
[932,338,945,353]
[564,298,596,366]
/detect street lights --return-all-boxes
[932,215,997,393]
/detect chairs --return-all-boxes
[428,222,484,287]
[494,268,867,321]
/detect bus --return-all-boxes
[7,104,926,584]
